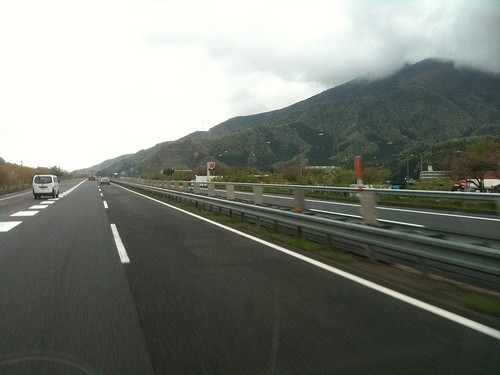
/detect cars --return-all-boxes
[100,177,110,185]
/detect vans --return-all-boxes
[32,175,60,199]
